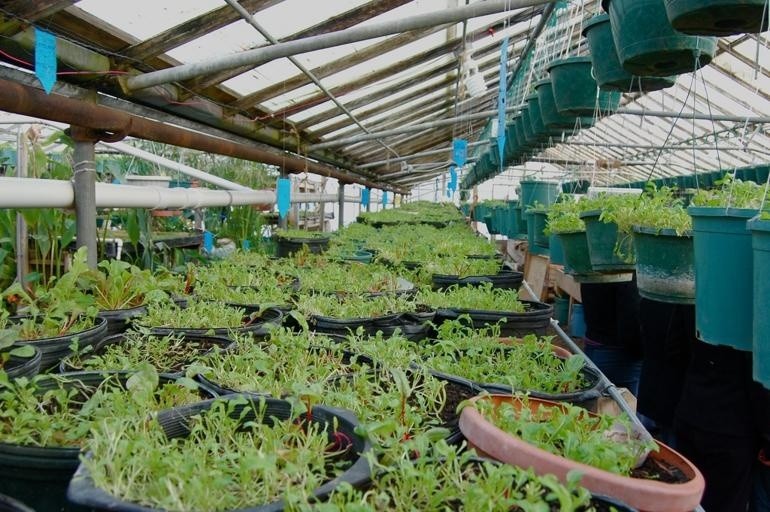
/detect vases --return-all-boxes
[500,0,769,144]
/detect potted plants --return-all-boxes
[0,145,769,512]
